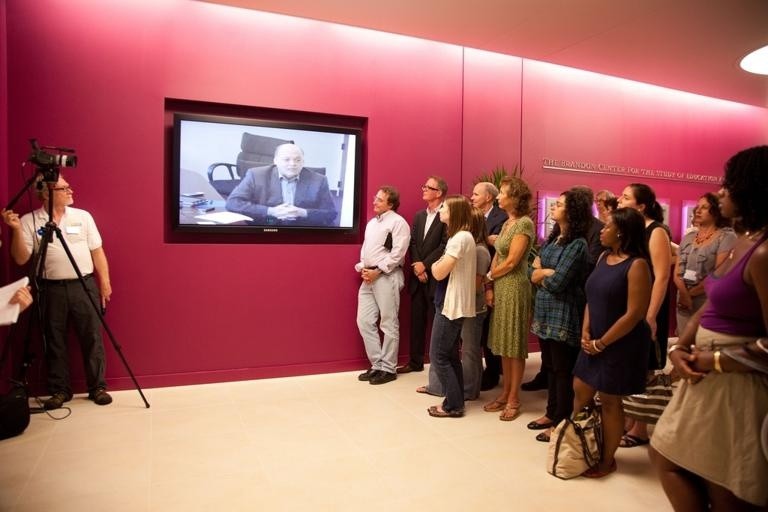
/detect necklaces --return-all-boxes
[727,226,766,259]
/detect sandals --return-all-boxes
[484,400,520,421]
[417,387,461,417]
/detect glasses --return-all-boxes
[54,187,70,191]
[422,185,438,190]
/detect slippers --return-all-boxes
[619,434,648,447]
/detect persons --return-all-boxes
[355,184,412,387]
[0,175,115,409]
[222,142,339,229]
[8,282,35,315]
[571,206,654,480]
[408,175,739,447]
[643,146,766,511]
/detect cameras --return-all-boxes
[29,139,78,182]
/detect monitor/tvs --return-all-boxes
[168,111,363,244]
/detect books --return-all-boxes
[0,274,31,326]
[181,192,204,198]
[180,197,209,208]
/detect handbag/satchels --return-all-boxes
[547,409,603,479]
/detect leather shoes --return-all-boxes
[528,421,556,441]
[521,379,547,390]
[584,460,617,477]
[359,363,424,384]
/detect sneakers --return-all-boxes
[44,393,65,409]
[89,391,112,404]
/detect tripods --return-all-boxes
[7,184,151,407]
